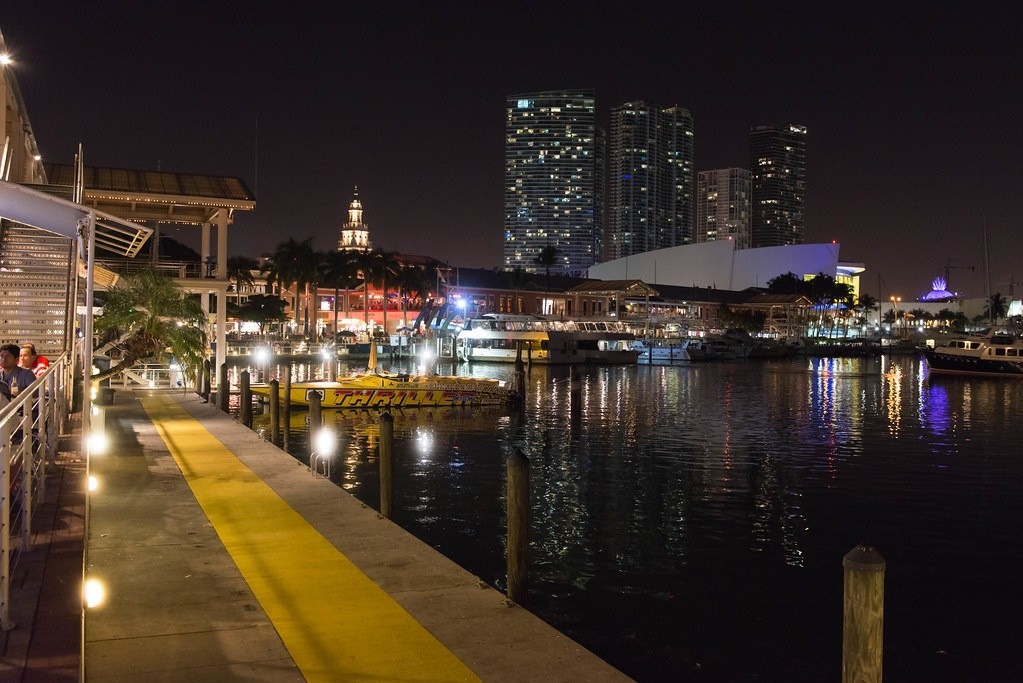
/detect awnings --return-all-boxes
[16,236,121,291]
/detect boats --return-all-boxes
[234,371,510,408]
[914,325,1023,379]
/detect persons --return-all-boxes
[0,393,19,434]
[17,343,48,381]
[0,344,39,396]
